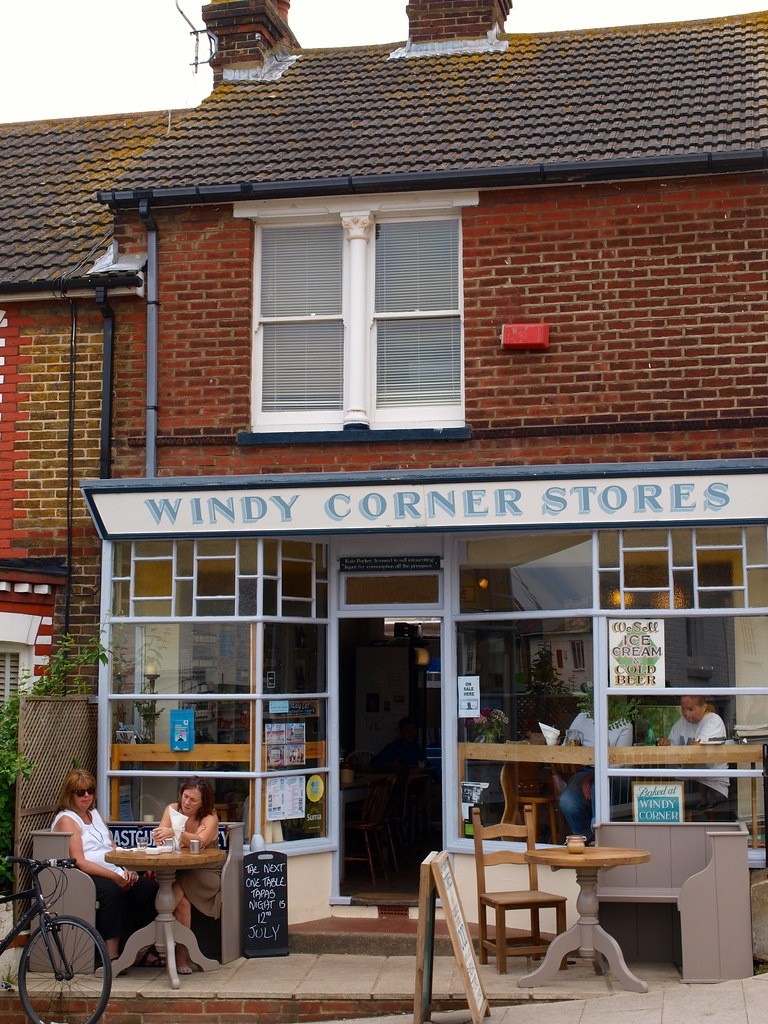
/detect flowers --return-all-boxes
[465,708,509,744]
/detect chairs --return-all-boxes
[472,804,568,975]
[346,750,379,774]
[345,775,397,887]
[500,739,565,846]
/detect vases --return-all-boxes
[485,730,496,744]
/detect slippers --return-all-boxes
[134,951,166,967]
[111,957,128,975]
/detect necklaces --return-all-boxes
[88,812,103,844]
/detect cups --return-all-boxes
[137,837,149,851]
[565,835,587,853]
[567,730,583,746]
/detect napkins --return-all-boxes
[538,722,560,745]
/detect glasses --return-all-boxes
[72,787,95,797]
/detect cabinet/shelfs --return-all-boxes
[140,623,250,821]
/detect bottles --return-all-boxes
[645,725,656,746]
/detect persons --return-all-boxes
[658,695,730,822]
[376,717,432,796]
[153,776,221,973]
[50,769,159,978]
[551,694,633,843]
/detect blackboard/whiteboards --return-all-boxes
[437,853,486,1013]
[243,851,288,958]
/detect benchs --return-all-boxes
[30,821,244,973]
[594,822,755,984]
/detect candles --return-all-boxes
[146,663,157,675]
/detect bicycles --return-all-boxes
[0,855,112,1024]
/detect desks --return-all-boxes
[339,774,385,884]
[517,846,652,993]
[458,740,766,848]
[95,848,226,989]
